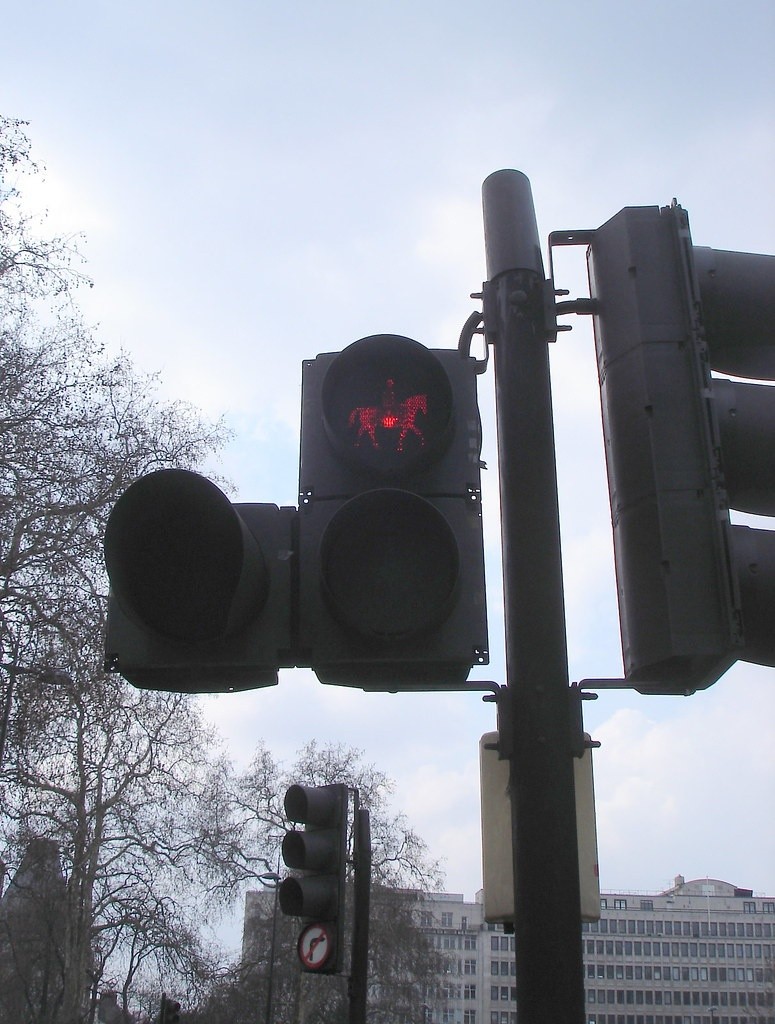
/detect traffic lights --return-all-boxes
[161,993,180,1024]
[99,468,292,698]
[281,332,495,499]
[291,486,492,689]
[275,783,349,920]
[587,196,774,695]
